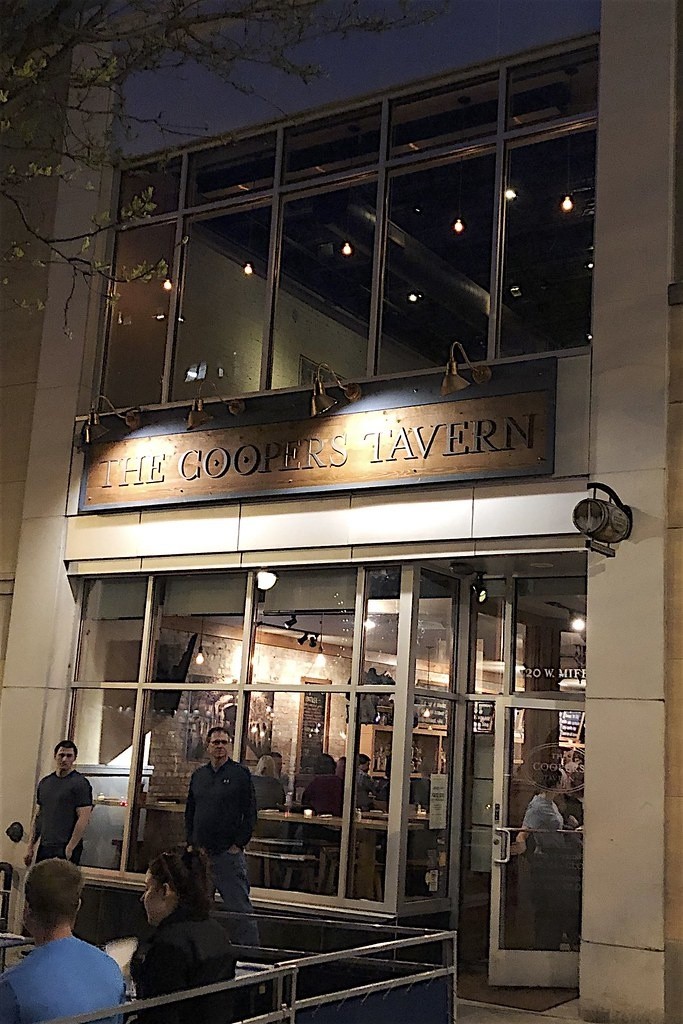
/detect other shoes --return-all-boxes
[19,949,33,958]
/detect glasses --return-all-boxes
[157,852,177,893]
[212,740,227,745]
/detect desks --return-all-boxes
[143,800,432,897]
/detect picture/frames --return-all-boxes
[297,354,348,388]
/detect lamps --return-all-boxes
[307,359,363,419]
[309,634,319,648]
[471,570,490,607]
[81,393,144,442]
[283,614,299,630]
[184,378,244,431]
[298,633,309,646]
[440,337,492,397]
[567,610,586,631]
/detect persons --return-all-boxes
[184,729,265,995]
[130,849,238,1024]
[235,741,449,898]
[13,740,95,955]
[510,761,585,952]
[0,858,125,1024]
